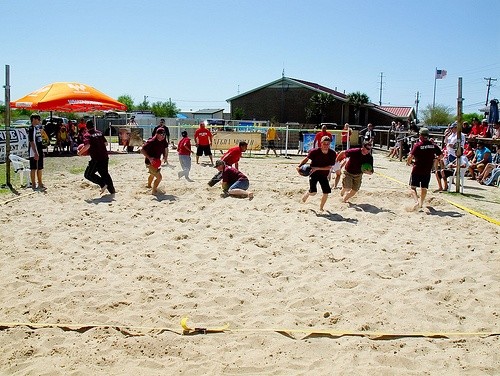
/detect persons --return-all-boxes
[26,112,500,213]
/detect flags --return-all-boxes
[436,70,448,79]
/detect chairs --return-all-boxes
[8,154,38,188]
[41,138,49,154]
[446,164,470,193]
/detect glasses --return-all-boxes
[160,122,164,123]
[365,146,370,150]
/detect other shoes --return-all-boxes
[33,187,38,190]
[39,185,47,189]
[152,191,159,195]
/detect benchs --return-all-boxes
[51,136,79,153]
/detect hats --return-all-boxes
[472,117,478,121]
[182,131,187,136]
[419,128,429,134]
[449,123,457,128]
[214,160,224,168]
[156,128,165,134]
[200,123,205,126]
[482,119,488,123]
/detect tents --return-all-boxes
[9,82,127,130]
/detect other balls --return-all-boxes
[298,166,311,177]
[77,144,88,156]
[361,163,372,172]
[152,159,161,168]
